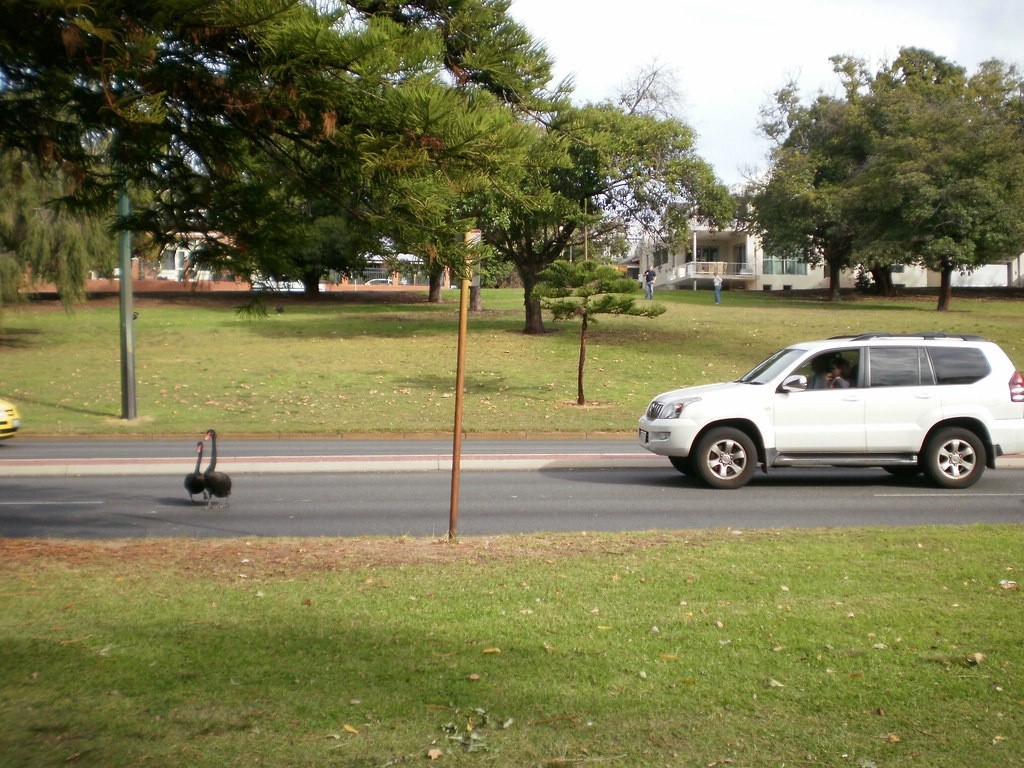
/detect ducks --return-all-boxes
[183,429,232,508]
[133,311,140,320]
[275,307,284,313]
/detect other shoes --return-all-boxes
[716,302,719,304]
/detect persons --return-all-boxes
[643,263,657,300]
[805,355,852,389]
[713,271,723,304]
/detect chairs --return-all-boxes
[836,358,850,377]
[697,262,727,275]
[848,364,858,387]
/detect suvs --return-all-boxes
[639,332,1024,490]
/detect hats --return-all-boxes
[713,271,718,274]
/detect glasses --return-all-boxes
[832,367,837,370]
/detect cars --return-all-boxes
[252,274,326,293]
[365,279,392,285]
[0,398,20,438]
